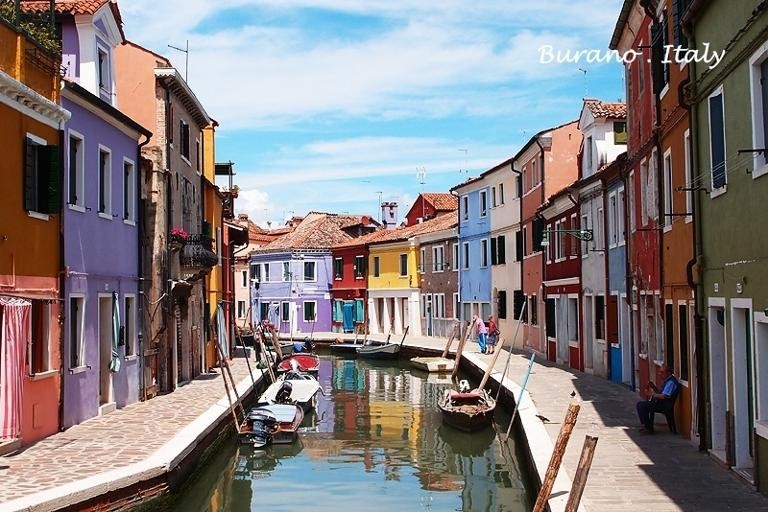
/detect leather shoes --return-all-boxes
[481,347,487,353]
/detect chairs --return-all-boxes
[646,383,682,434]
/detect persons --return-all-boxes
[486,315,497,355]
[473,315,487,353]
[254,330,261,361]
[637,366,679,432]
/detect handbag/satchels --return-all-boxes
[494,329,500,343]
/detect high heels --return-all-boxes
[485,351,494,355]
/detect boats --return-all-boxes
[356,344,402,360]
[410,356,455,372]
[258,369,319,412]
[276,353,321,378]
[238,402,305,448]
[437,387,497,433]
[235,331,294,353]
[330,343,364,352]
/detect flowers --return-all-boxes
[171,227,188,243]
